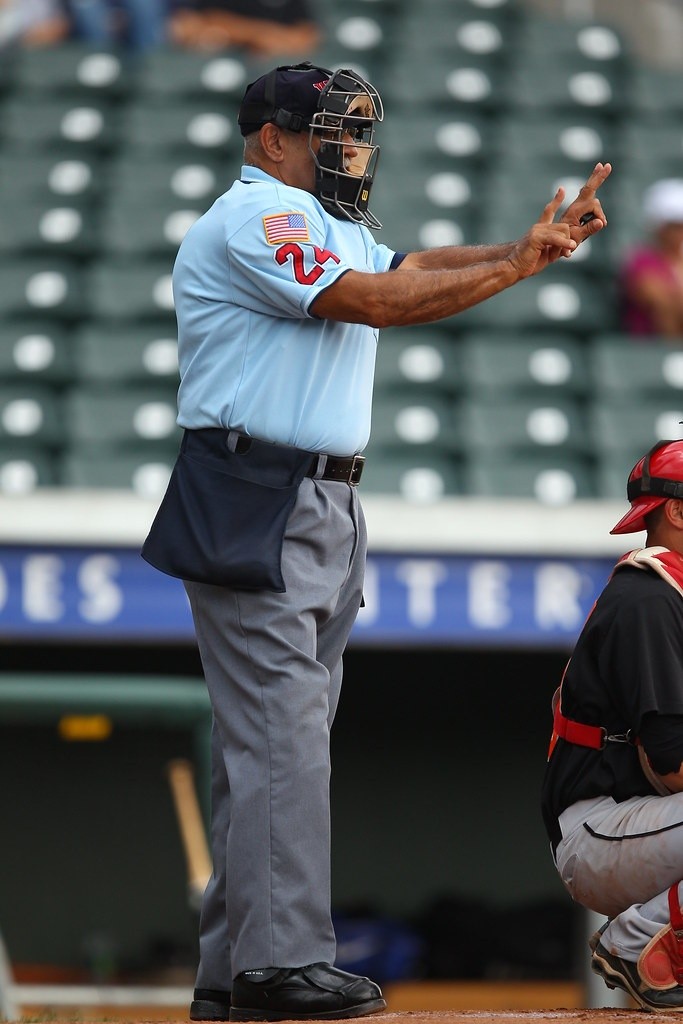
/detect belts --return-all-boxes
[196,427,365,486]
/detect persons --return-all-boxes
[609,177,681,340]
[543,435,683,1010]
[141,57,613,1021]
[2,1,321,57]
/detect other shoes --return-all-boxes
[589,919,682,1013]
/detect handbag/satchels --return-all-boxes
[140,428,313,593]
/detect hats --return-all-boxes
[237,61,373,137]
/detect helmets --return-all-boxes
[610,440,683,534]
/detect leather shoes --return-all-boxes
[190,988,231,1020]
[230,960,387,1020]
